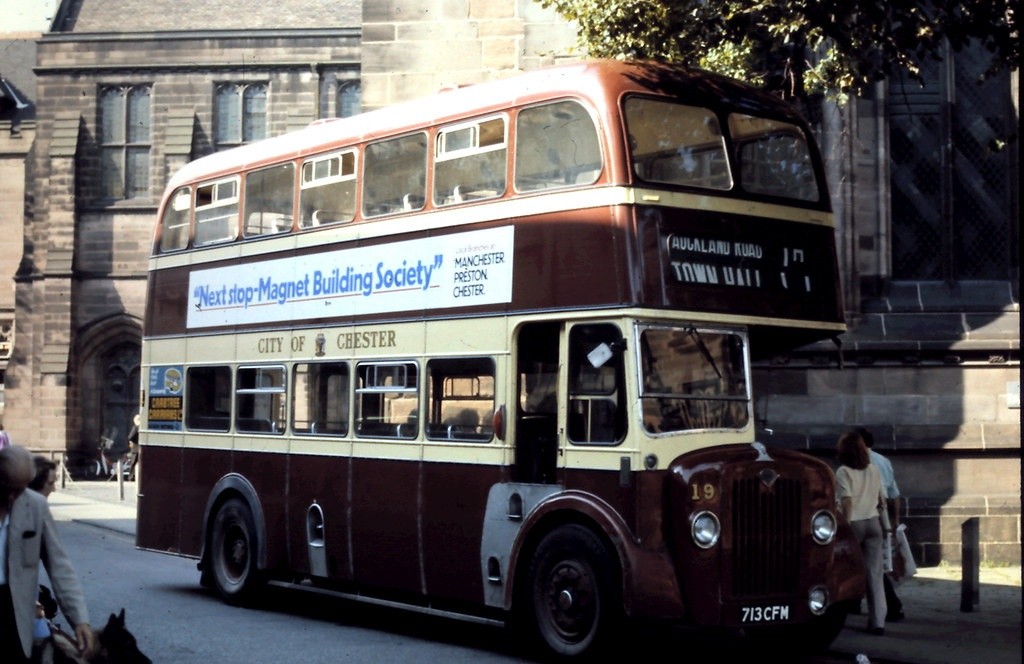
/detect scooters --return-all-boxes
[84,436,140,484]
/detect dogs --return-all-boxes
[87,608,153,664]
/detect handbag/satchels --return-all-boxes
[886,523,917,590]
[37,583,57,620]
[882,533,893,573]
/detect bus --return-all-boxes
[133,59,865,664]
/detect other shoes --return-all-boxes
[886,598,902,622]
[867,628,885,636]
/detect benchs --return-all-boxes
[186,416,271,432]
[271,419,491,440]
[272,179,570,233]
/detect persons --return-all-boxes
[835,427,903,636]
[0,424,94,664]
[128,414,140,508]
[407,408,492,433]
